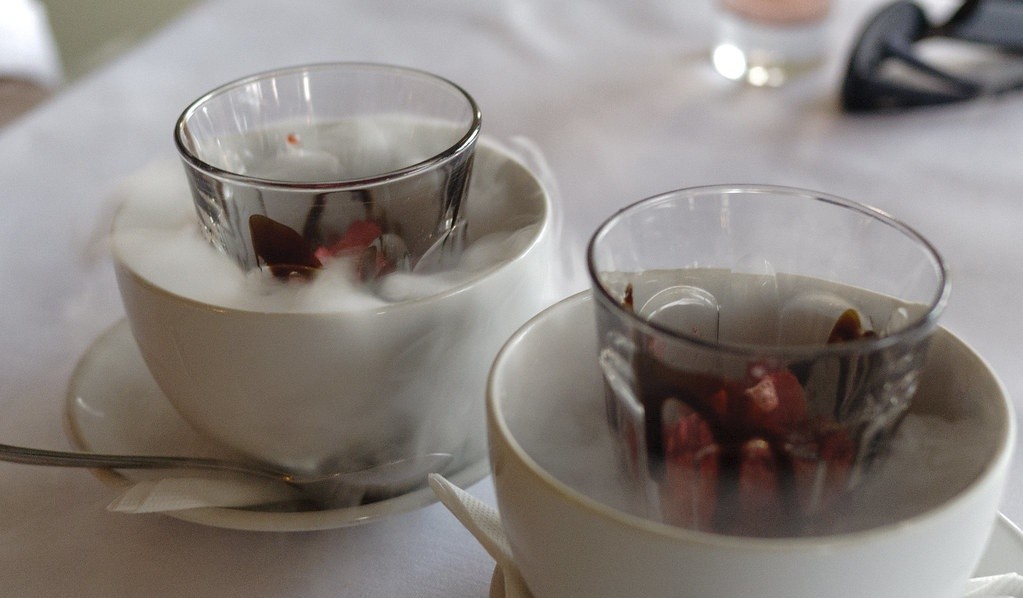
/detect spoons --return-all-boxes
[0,442,454,495]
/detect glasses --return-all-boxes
[840,0,1023,113]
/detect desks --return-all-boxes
[0,2,1023,597]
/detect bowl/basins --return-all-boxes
[484,287,1016,598]
[111,117,559,496]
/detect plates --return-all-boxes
[487,510,1021,598]
[62,314,493,531]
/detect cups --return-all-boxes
[710,0,834,84]
[172,62,482,282]
[586,182,950,524]
